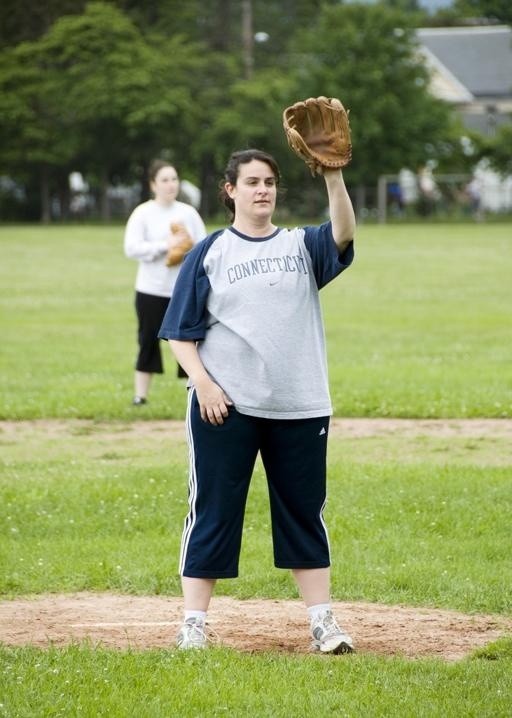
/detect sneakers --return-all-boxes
[176,618,209,650]
[310,610,354,653]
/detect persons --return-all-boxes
[1,148,489,224]
[123,158,207,407]
[157,151,356,657]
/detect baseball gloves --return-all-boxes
[283,95,354,176]
[166,224,196,265]
